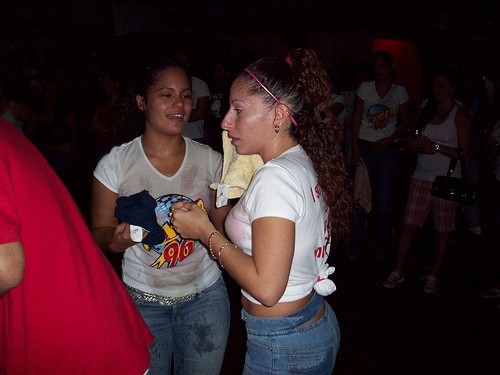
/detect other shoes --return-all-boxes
[469,226,482,235]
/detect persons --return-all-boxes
[0,114,155,375]
[89,56,232,375]
[169,48,359,375]
[1,47,254,173]
[321,50,500,299]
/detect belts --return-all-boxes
[126,286,199,305]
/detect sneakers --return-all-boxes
[424,275,438,294]
[382,272,405,288]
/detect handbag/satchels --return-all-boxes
[431,152,478,205]
[354,161,373,214]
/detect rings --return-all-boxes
[169,207,174,217]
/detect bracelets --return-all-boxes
[431,142,441,154]
[217,243,243,270]
[208,230,223,260]
